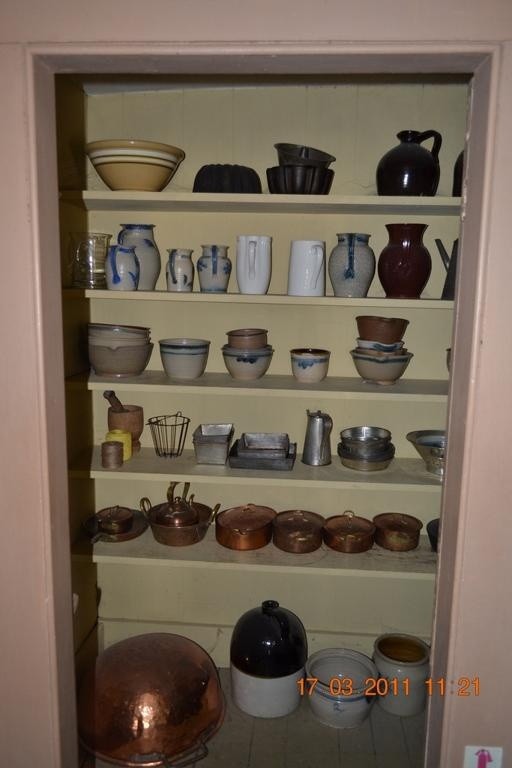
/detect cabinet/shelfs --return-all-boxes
[81,188,464,582]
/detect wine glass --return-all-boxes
[107,405,142,451]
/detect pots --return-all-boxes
[320,509,379,555]
[371,510,423,551]
[92,499,146,547]
[216,503,277,555]
[78,630,226,767]
[139,494,220,545]
[270,508,324,553]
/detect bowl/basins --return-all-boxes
[154,336,211,382]
[406,428,447,478]
[348,313,414,383]
[273,138,335,165]
[80,136,188,191]
[289,347,331,385]
[85,322,154,381]
[189,418,297,472]
[338,425,394,472]
[222,328,274,383]
[191,162,262,190]
[265,167,336,193]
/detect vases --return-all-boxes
[374,126,445,199]
[164,245,197,293]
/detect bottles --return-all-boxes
[230,599,307,719]
[163,247,193,292]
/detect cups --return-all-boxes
[285,239,327,297]
[105,428,133,460]
[234,234,273,295]
[101,442,124,467]
[73,230,112,291]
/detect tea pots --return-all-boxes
[300,410,334,467]
[435,235,457,299]
[377,129,443,194]
[157,482,199,527]
